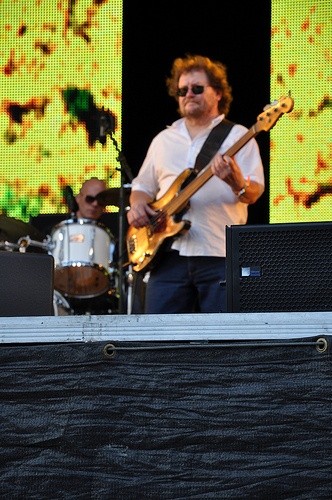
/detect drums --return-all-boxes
[45,218,116,299]
[74,270,125,311]
[53,289,73,316]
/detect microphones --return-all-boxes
[99,106,106,137]
[65,187,79,223]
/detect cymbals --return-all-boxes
[0,215,40,238]
[96,187,131,206]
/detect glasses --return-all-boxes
[176,85,215,97]
[78,195,97,204]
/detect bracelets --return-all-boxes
[232,173,251,198]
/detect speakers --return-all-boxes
[0,251,57,317]
[225,221,332,314]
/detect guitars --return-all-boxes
[126,92,294,271]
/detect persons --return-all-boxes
[128,55,264,315]
[76,178,108,224]
